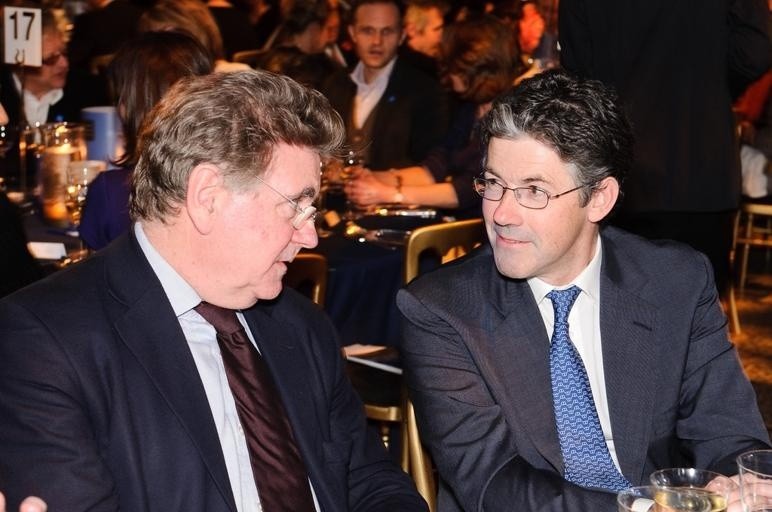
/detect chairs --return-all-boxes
[339,219,484,447]
[287,252,327,311]
[728,71,772,292]
[402,400,434,511]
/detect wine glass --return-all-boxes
[65,160,102,264]
[315,157,331,214]
[334,149,365,220]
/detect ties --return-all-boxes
[189,292,319,512]
[539,286,643,500]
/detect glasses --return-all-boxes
[471,164,597,211]
[245,167,319,231]
[42,47,70,69]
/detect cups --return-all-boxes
[17,118,88,225]
[615,448,772,511]
[80,105,120,167]
